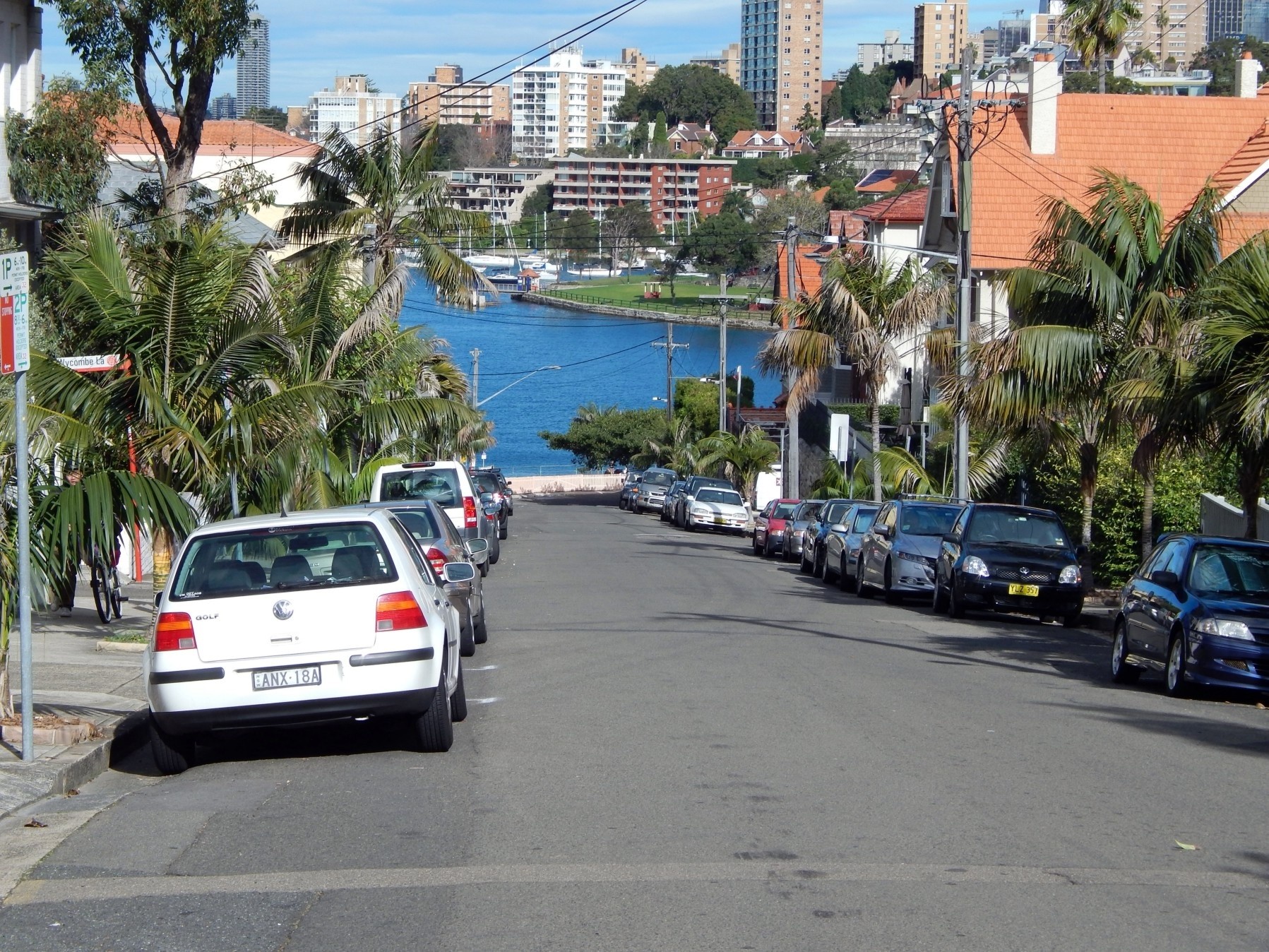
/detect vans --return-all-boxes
[368,459,494,578]
[619,467,734,528]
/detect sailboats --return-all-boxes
[394,175,711,294]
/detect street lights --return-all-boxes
[469,365,562,470]
[822,234,972,505]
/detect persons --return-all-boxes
[52,462,123,616]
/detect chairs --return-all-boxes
[330,545,381,577]
[239,561,268,587]
[396,514,428,537]
[269,555,315,585]
[207,560,250,591]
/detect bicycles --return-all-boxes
[90,553,129,624]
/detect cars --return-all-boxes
[682,486,752,538]
[1110,533,1269,703]
[145,506,478,774]
[283,496,490,658]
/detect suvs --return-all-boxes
[752,498,805,558]
[854,492,980,606]
[820,502,885,593]
[781,499,828,563]
[931,501,1089,628]
[438,465,514,540]
[798,498,871,578]
